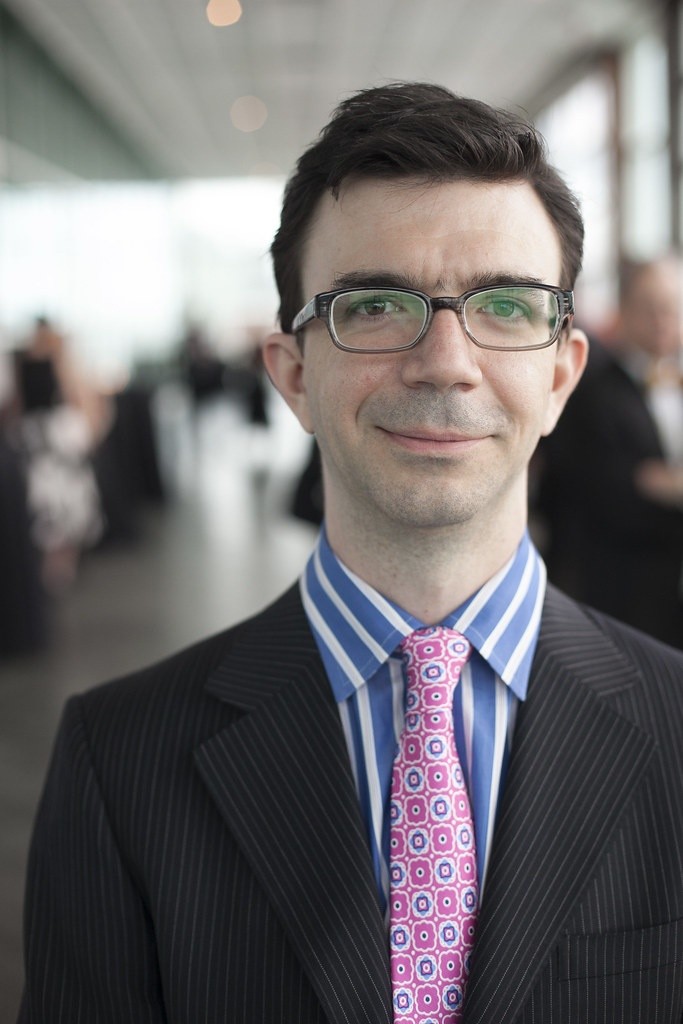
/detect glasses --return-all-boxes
[291,282,575,354]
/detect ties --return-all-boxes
[388,627,480,1024]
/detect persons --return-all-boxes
[0,82,683,1024]
